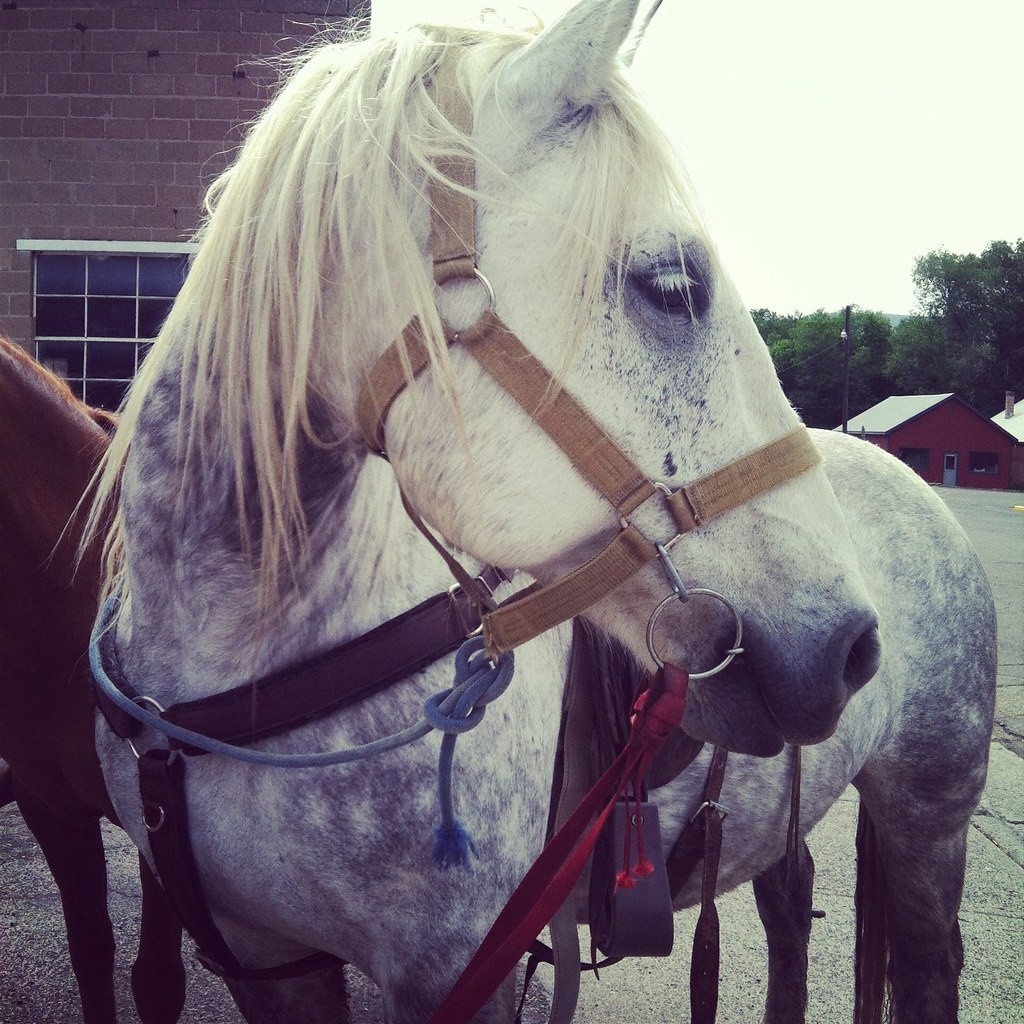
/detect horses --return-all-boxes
[47,0,1001,1024]
